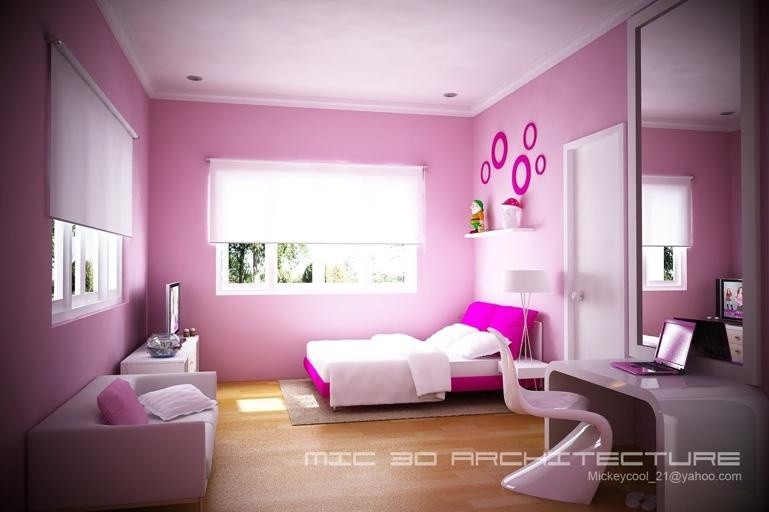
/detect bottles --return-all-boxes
[190,327,196,336]
[183,328,189,337]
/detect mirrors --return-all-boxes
[628,2,760,387]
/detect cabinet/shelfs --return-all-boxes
[119,333,200,375]
[725,324,744,365]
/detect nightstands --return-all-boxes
[497,357,549,415]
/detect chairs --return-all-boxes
[486,328,613,506]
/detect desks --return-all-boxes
[542,358,769,512]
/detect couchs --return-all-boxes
[23,369,218,511]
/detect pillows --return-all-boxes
[424,322,479,353]
[453,331,512,359]
[138,382,218,422]
[96,378,149,425]
[461,301,540,360]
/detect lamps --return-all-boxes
[504,269,552,365]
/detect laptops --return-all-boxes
[610,318,697,375]
[674,317,733,363]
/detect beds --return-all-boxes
[303,320,544,413]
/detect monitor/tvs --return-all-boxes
[715,277,744,326]
[166,282,186,347]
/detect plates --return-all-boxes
[146,345,182,358]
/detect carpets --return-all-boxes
[278,375,514,426]
